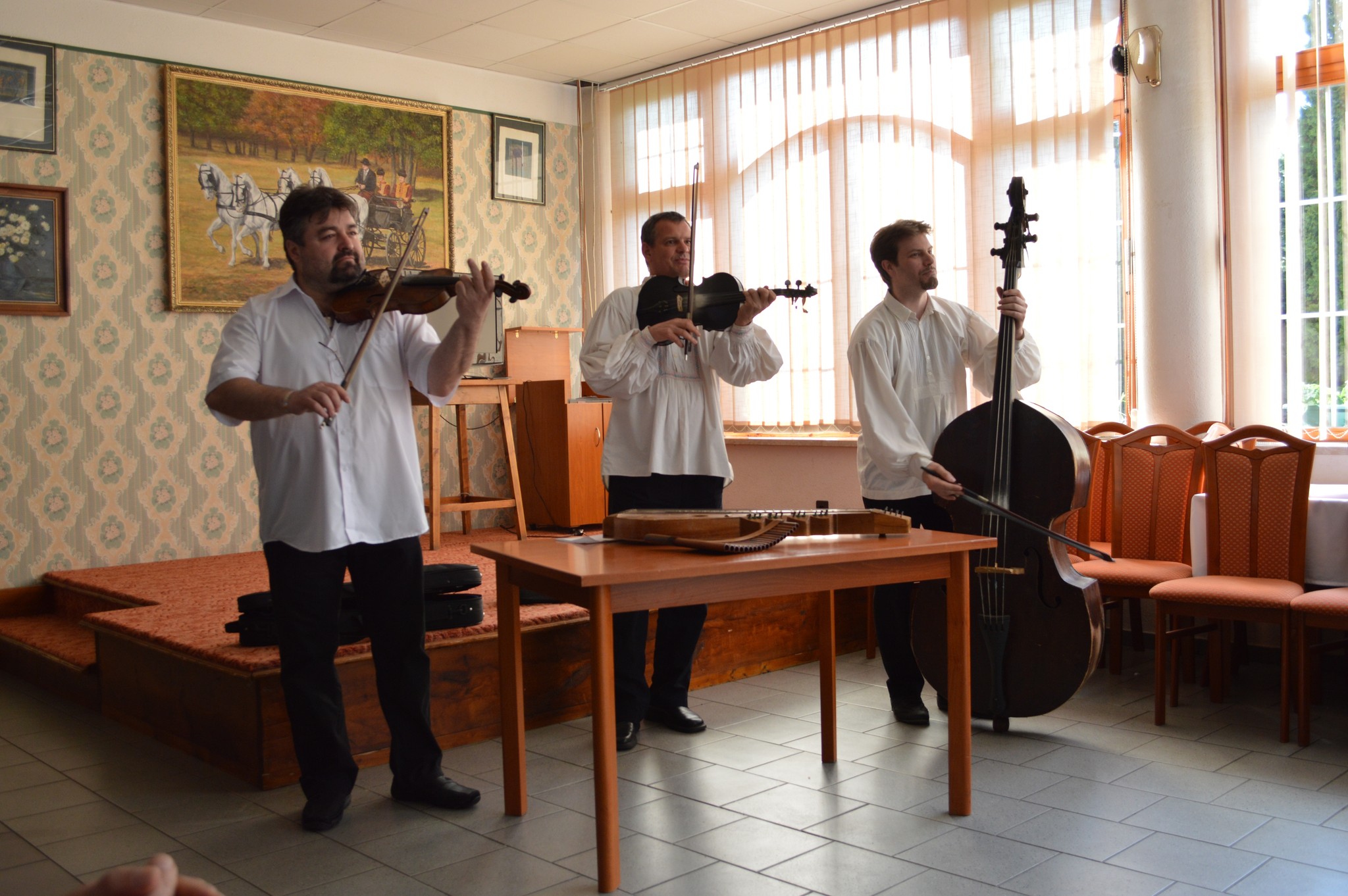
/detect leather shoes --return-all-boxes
[390,775,481,810]
[614,721,641,749]
[646,706,707,733]
[890,694,930,726]
[301,792,354,832]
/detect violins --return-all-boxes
[320,266,532,327]
[635,272,818,346]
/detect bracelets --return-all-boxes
[284,391,305,416]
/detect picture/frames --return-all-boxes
[164,62,456,314]
[0,182,71,316]
[492,114,546,206]
[0,35,56,153]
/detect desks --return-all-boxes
[410,377,526,551]
[470,525,999,893]
[1188,481,1348,588]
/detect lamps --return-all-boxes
[1126,25,1164,87]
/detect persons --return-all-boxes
[387,170,411,206]
[375,167,390,196]
[74,852,225,896]
[847,221,1041,728]
[207,187,492,833]
[581,211,784,749]
[354,158,375,191]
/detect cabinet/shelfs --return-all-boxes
[519,380,611,527]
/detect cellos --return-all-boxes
[910,176,1107,731]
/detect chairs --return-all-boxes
[1065,422,1348,750]
